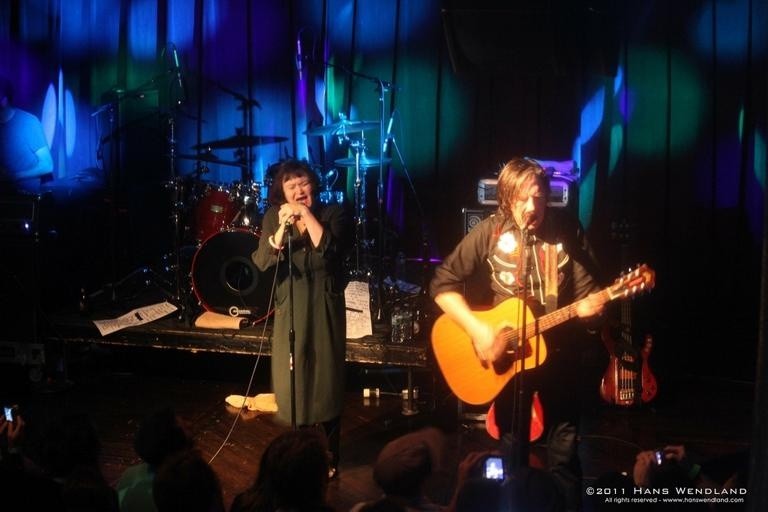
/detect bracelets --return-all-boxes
[269,235,285,250]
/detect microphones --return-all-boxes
[170,45,185,86]
[284,200,307,231]
[520,212,538,233]
[298,31,303,81]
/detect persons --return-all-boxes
[430,157,608,512]
[0,408,749,512]
[250,158,346,481]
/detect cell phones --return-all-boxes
[481,456,505,481]
[4,406,17,428]
[654,448,666,467]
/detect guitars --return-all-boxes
[600,262,658,407]
[431,264,654,405]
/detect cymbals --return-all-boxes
[192,136,287,149]
[302,119,380,135]
[333,157,391,167]
[178,153,240,168]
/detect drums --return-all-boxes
[192,179,260,243]
[190,227,276,325]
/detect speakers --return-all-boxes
[0,192,49,365]
[463,209,577,315]
